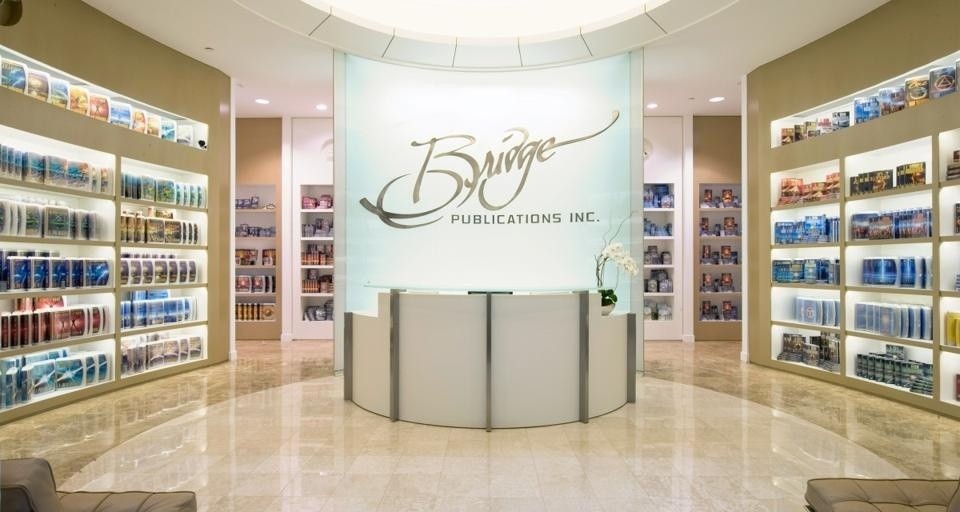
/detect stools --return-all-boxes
[804,478,960,512]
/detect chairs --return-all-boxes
[0,456,198,512]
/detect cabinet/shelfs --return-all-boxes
[0,42,230,426]
[640,174,685,340]
[232,155,284,342]
[745,56,960,421]
[285,172,344,342]
[691,173,748,343]
